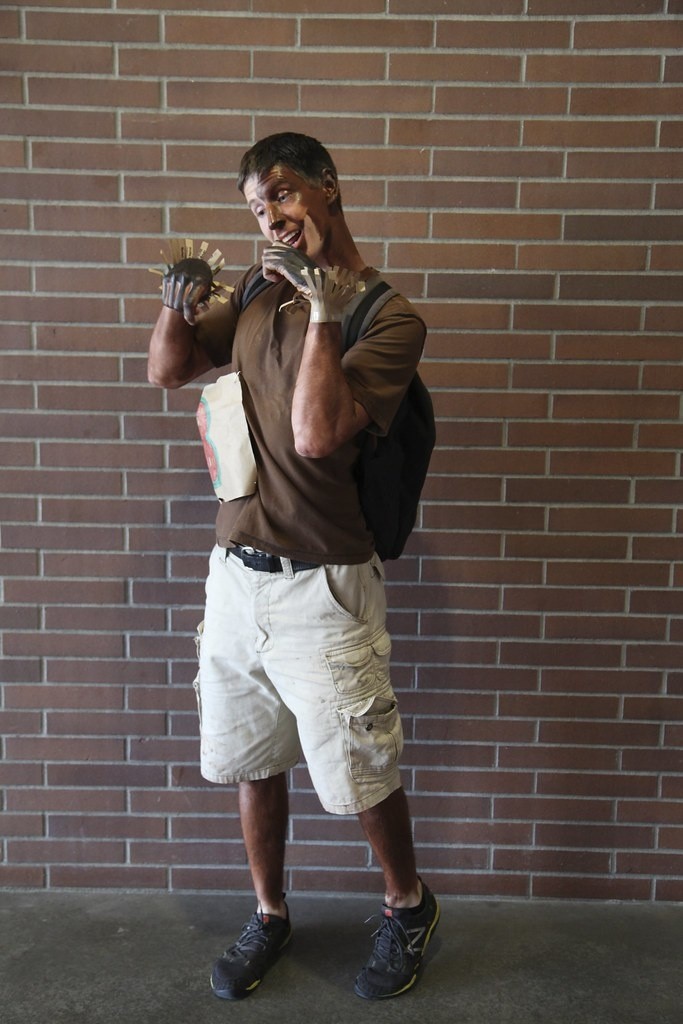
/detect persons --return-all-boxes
[148,132,440,1001]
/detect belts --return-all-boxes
[223,537,323,574]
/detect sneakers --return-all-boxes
[209,891,295,1001]
[351,872,444,1002]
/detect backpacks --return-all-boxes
[237,258,438,565]
